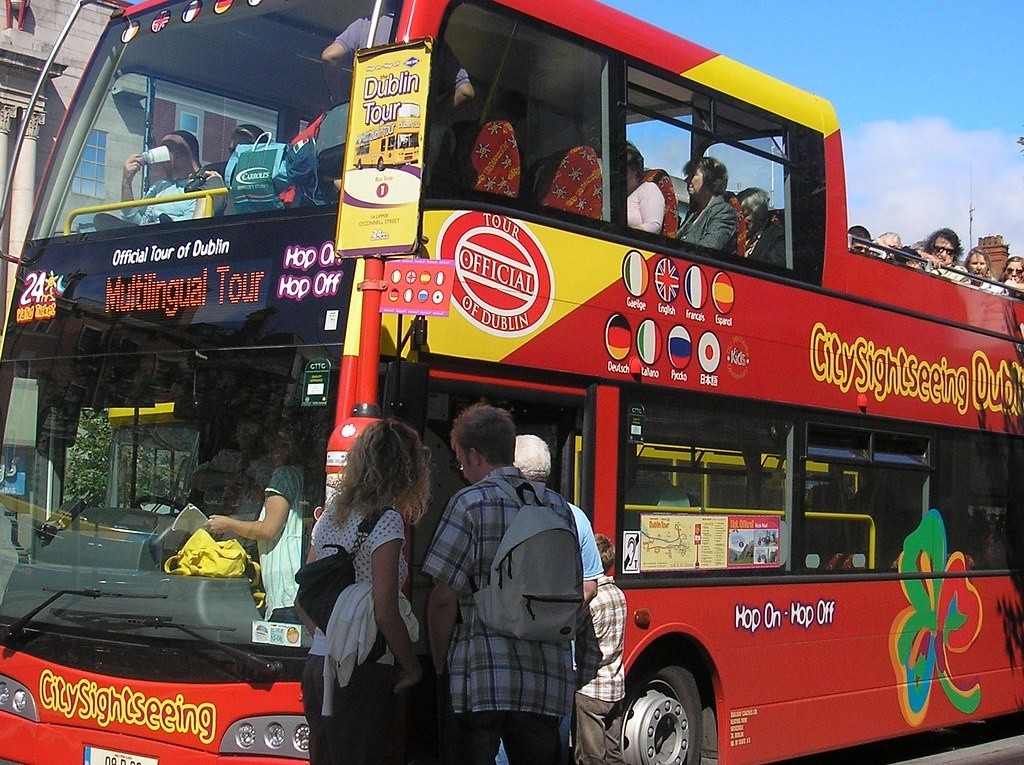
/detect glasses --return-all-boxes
[854,246,869,252]
[452,448,481,471]
[1007,267,1024,274]
[934,246,955,257]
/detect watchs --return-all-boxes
[223,471,234,485]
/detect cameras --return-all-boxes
[183,166,212,193]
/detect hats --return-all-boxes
[191,449,241,490]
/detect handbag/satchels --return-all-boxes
[164,528,261,585]
[225,132,296,214]
[295,507,397,665]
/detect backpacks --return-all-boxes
[469,477,586,644]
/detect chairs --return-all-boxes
[818,529,1008,570]
[466,117,748,260]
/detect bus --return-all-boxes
[2,1,1024,765]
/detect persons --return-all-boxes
[495,434,627,765]
[805,457,987,563]
[295,418,431,765]
[94,0,475,232]
[849,226,1024,301]
[625,141,786,268]
[190,409,472,624]
[421,403,583,765]
